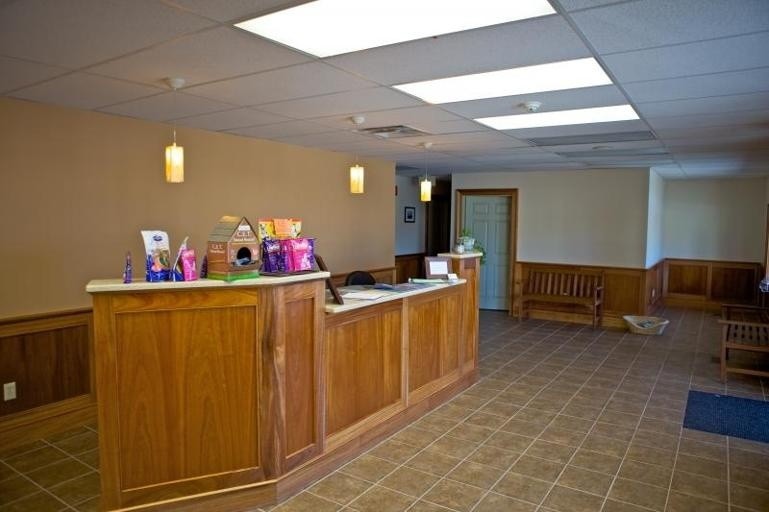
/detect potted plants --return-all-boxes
[457,227,486,266]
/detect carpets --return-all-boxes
[682,382,769,448]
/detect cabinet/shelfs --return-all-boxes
[85,252,482,512]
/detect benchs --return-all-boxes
[515,269,606,330]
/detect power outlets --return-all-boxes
[2,381,17,402]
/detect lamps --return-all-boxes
[349,116,367,196]
[418,142,435,203]
[162,76,187,185]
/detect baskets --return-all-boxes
[623,315,670,335]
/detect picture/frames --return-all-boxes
[404,207,415,223]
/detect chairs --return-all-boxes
[717,300,769,384]
